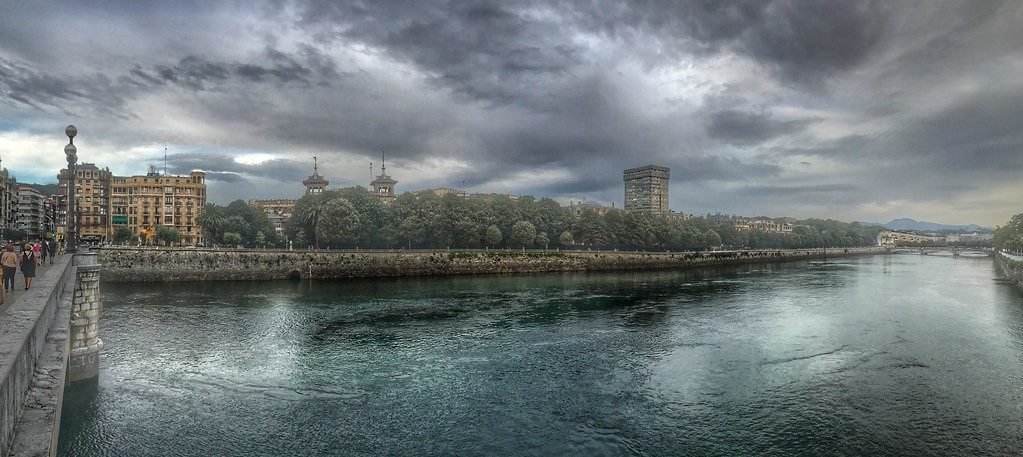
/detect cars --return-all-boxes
[195,241,205,247]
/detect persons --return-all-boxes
[0,236,67,293]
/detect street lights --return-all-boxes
[65,125,79,253]
[285,234,288,251]
[104,212,107,248]
